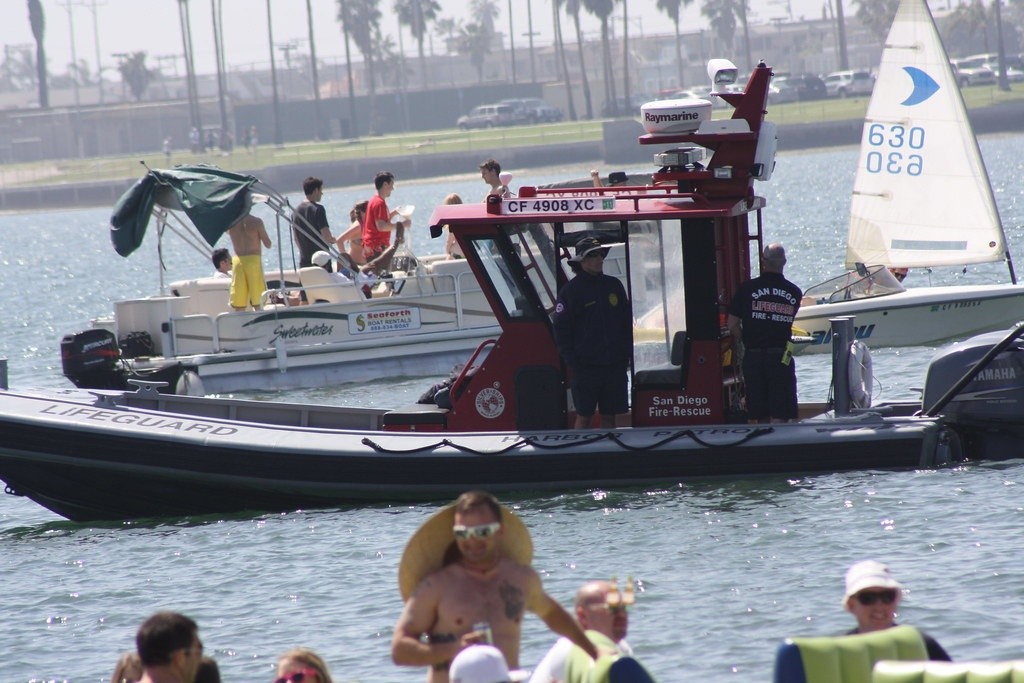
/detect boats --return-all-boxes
[0,55,1024,517]
[92,1,1024,390]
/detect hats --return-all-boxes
[843,561,902,612]
[397,501,535,607]
[449,645,529,683]
[608,172,628,184]
[567,237,612,270]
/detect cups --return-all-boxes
[472,623,493,645]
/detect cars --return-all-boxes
[456,97,562,129]
[601,51,1023,118]
[191,126,231,151]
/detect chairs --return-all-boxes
[772,623,1024,683]
[299,266,354,304]
[636,332,687,386]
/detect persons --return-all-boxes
[830,559,953,662]
[275,649,334,683]
[525,579,635,683]
[726,243,799,424]
[606,171,629,187]
[110,612,222,683]
[391,491,617,683]
[888,267,908,284]
[448,644,529,683]
[213,158,510,313]
[555,236,632,431]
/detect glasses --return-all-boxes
[452,523,501,541]
[584,601,632,614]
[275,669,316,683]
[892,268,906,278]
[852,590,896,605]
[588,250,606,257]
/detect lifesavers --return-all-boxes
[847,339,875,409]
